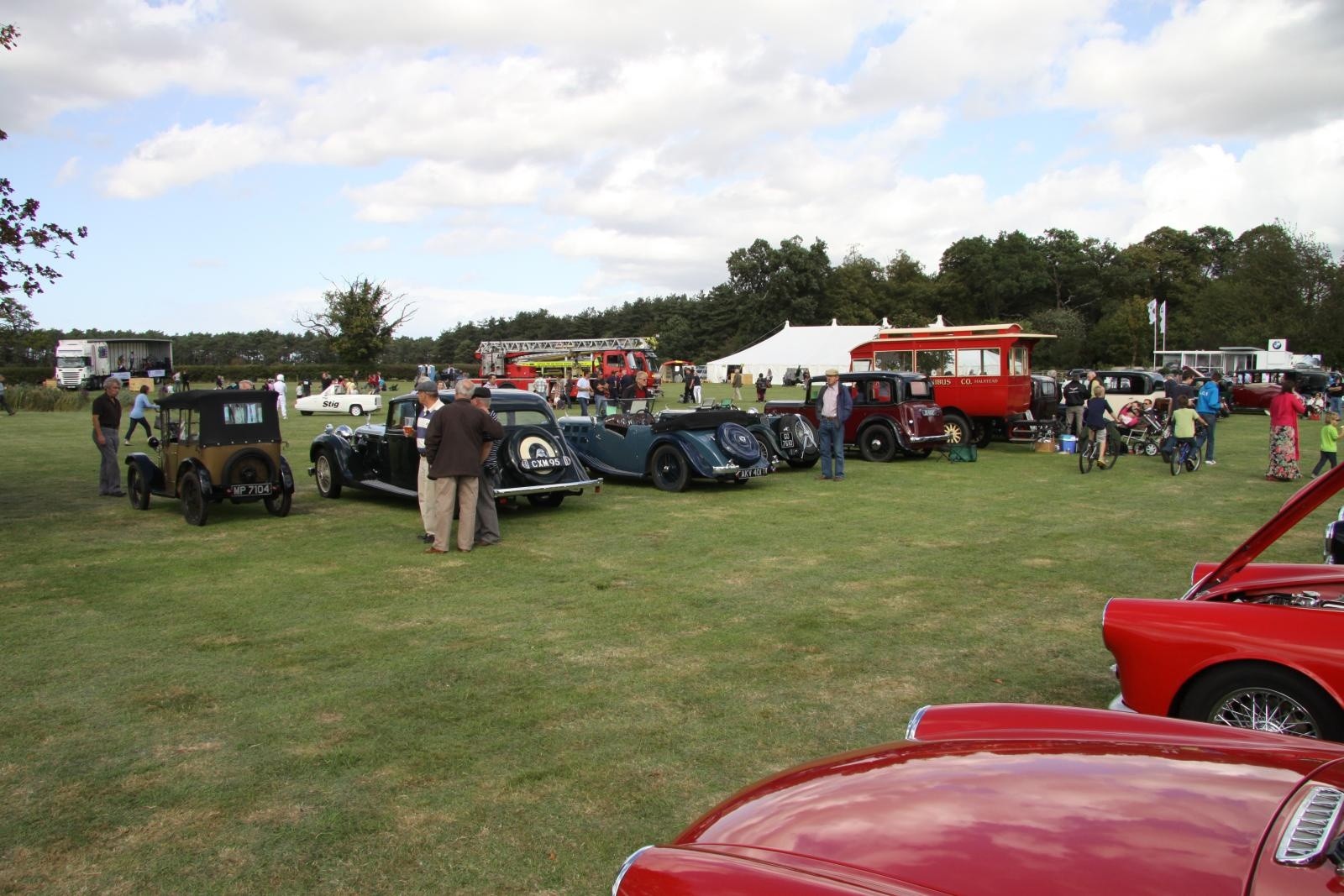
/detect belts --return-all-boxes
[822,416,838,420]
[420,452,427,457]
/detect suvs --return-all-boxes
[765,371,952,463]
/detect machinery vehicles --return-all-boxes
[468,337,665,406]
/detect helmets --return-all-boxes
[277,374,285,382]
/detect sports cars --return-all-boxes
[1102,456,1344,750]
[559,394,818,489]
[611,703,1344,896]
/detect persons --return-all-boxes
[1085,386,1121,467]
[1032,342,1344,453]
[815,369,853,482]
[1170,394,1209,468]
[1196,370,1226,466]
[122,384,160,446]
[463,386,504,547]
[1264,379,1306,480]
[1310,410,1344,478]
[421,378,504,555]
[91,377,126,497]
[402,381,446,545]
[51,342,821,430]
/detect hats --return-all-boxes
[413,381,438,392]
[1066,370,1074,377]
[470,387,491,399]
[825,368,838,376]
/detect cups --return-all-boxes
[404,417,414,438]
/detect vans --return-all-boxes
[849,322,1058,455]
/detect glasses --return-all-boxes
[1334,419,1340,422]
[1142,403,1150,405]
[416,393,420,396]
[639,378,648,381]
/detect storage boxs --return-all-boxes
[1034,442,1056,453]
[1059,434,1077,454]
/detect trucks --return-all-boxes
[56,339,174,390]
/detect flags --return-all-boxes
[1159,303,1164,334]
[1147,299,1156,325]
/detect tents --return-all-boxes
[703,315,1026,385]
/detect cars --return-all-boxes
[123,386,295,524]
[307,389,602,521]
[294,384,382,417]
[1026,329,1344,445]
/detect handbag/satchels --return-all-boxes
[947,443,978,462]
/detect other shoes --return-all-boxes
[1097,457,1108,467]
[417,534,434,544]
[123,438,130,445]
[1205,460,1216,465]
[833,475,845,482]
[473,538,490,546]
[283,416,289,419]
[1186,457,1195,469]
[1309,472,1318,479]
[104,491,126,497]
[816,474,833,480]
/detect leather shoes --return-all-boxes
[423,546,445,554]
